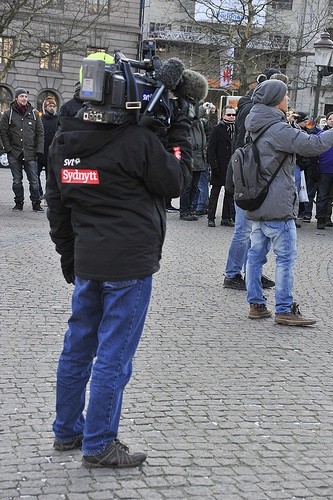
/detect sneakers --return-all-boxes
[275,303,317,325]
[248,303,272,319]
[244,273,275,288]
[222,274,247,291]
[54,435,83,450]
[82,438,147,468]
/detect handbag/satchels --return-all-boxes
[297,170,309,202]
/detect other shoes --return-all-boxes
[13,202,24,211]
[43,198,48,207]
[295,221,301,228]
[297,214,303,219]
[325,219,333,228]
[303,215,311,222]
[164,206,235,227]
[317,221,325,229]
[32,203,44,212]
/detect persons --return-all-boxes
[286,106,333,230]
[37,96,61,207]
[59,82,87,126]
[163,102,236,227]
[223,68,289,292]
[0,87,46,212]
[46,51,193,469]
[243,77,333,327]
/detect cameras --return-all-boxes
[207,104,213,108]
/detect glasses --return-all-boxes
[225,113,236,116]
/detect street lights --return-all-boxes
[312,28,333,121]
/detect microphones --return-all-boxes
[146,57,185,111]
[175,69,208,104]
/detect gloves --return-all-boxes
[177,99,195,119]
[36,152,43,162]
[61,255,77,286]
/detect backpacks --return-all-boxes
[231,122,294,211]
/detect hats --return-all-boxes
[295,112,308,123]
[257,69,280,83]
[15,88,27,98]
[75,82,81,91]
[44,96,56,108]
[78,51,115,85]
[251,78,287,106]
